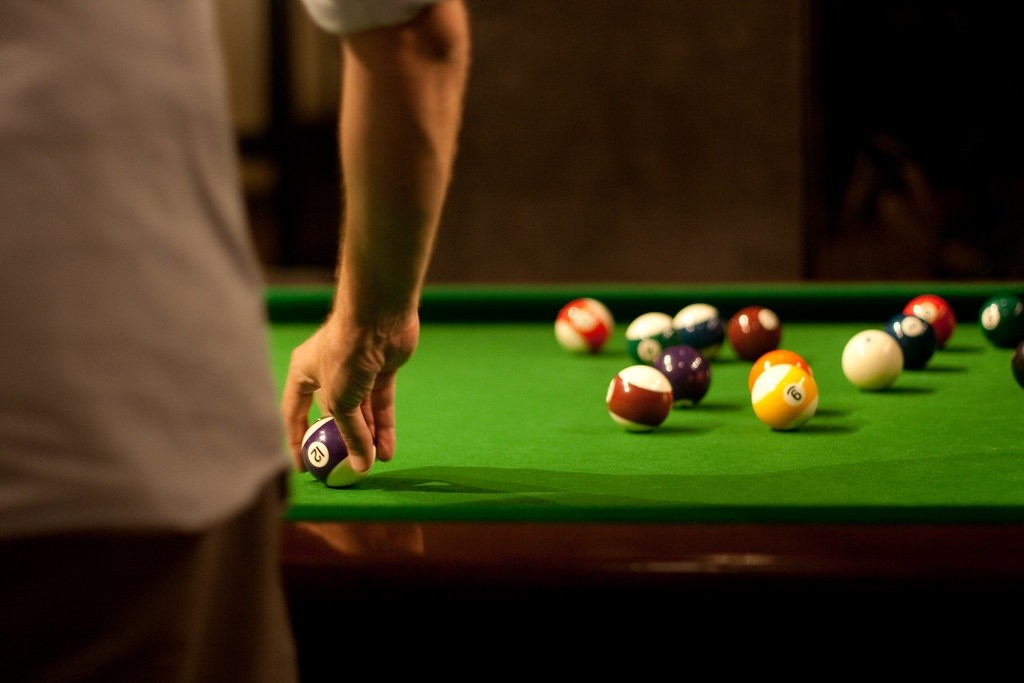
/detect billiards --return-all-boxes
[299,413,379,489]
[552,291,1024,435]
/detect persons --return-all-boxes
[0,0,469,683]
